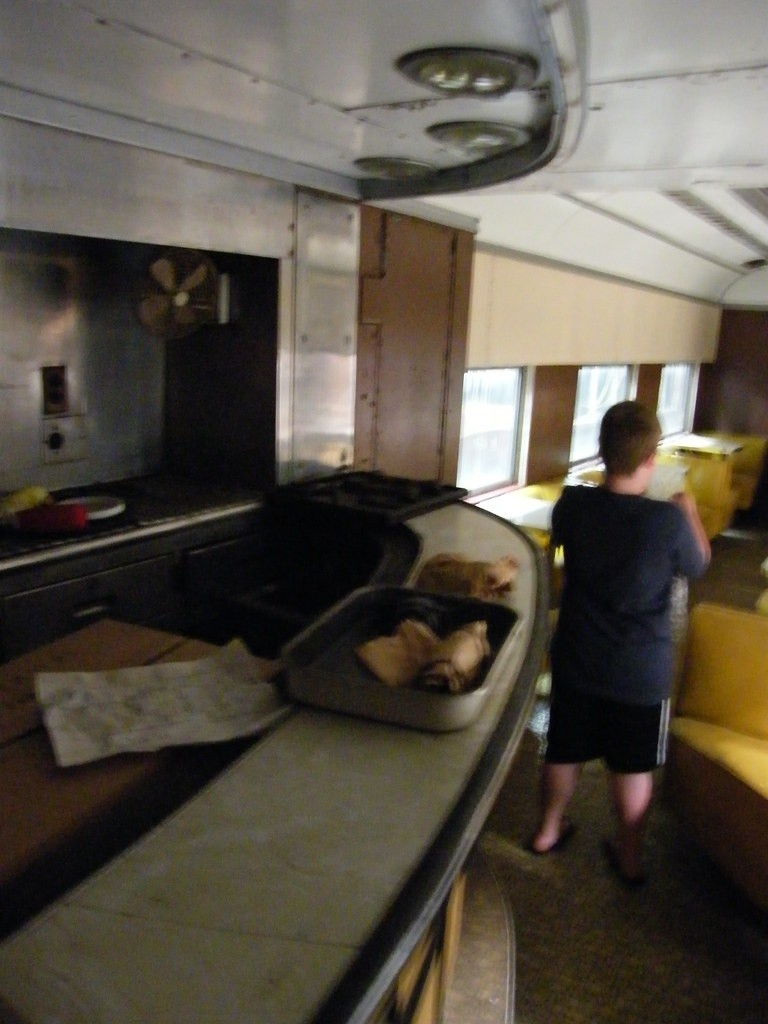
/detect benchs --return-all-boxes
[665,603,768,913]
[656,454,727,540]
[688,432,766,510]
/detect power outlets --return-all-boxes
[42,419,85,463]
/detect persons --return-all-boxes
[528,401,714,878]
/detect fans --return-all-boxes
[133,246,219,340]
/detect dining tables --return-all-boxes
[474,492,556,532]
[670,434,743,455]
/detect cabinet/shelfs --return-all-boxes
[0,512,268,665]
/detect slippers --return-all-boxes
[529,816,577,851]
[606,833,645,886]
